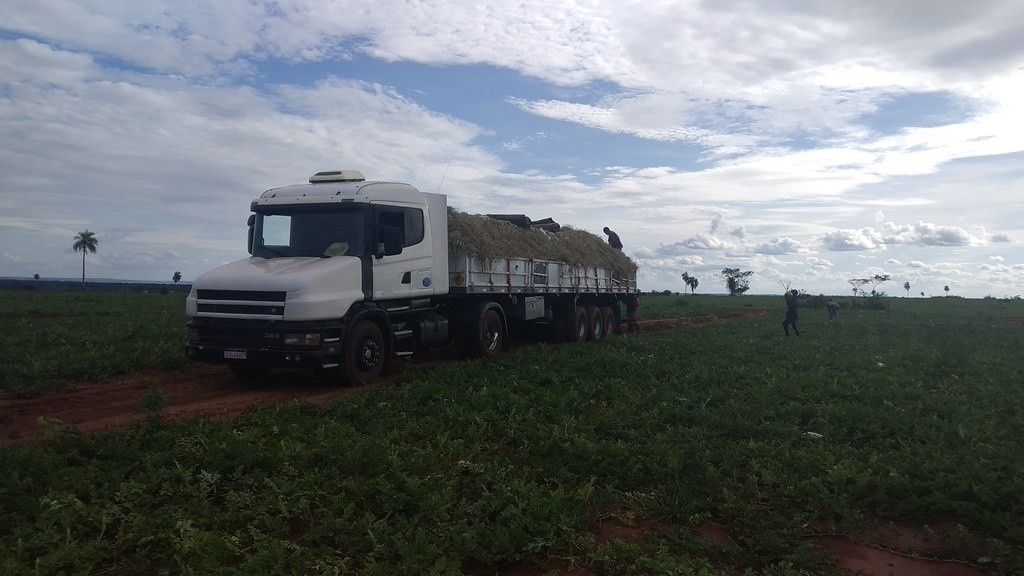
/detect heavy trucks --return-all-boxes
[184,168,642,383]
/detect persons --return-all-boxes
[621,293,640,334]
[603,227,623,251]
[783,290,800,336]
[828,302,841,320]
